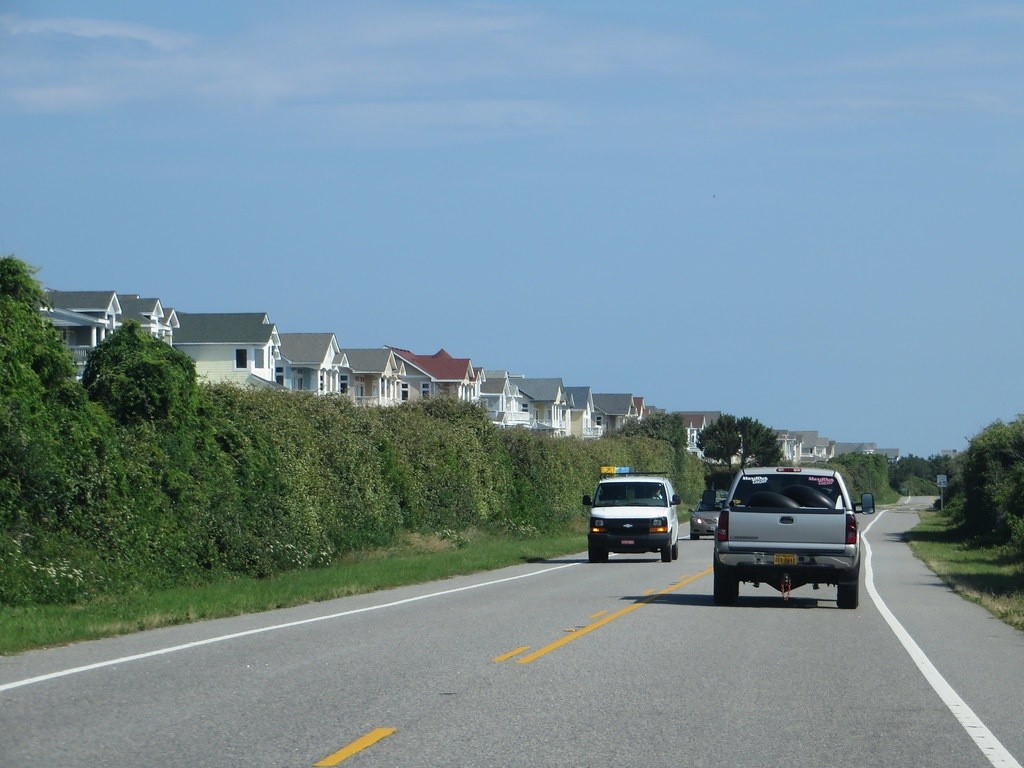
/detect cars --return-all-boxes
[688,498,723,540]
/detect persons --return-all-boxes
[650,486,665,499]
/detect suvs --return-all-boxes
[582,471,681,562]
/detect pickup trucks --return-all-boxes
[699,466,875,609]
